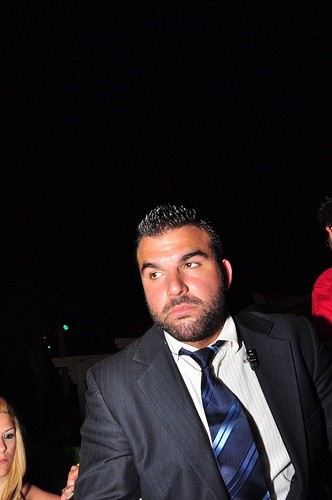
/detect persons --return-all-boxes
[0,399,79,500]
[76,204,332,500]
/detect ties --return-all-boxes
[178,340,272,500]
